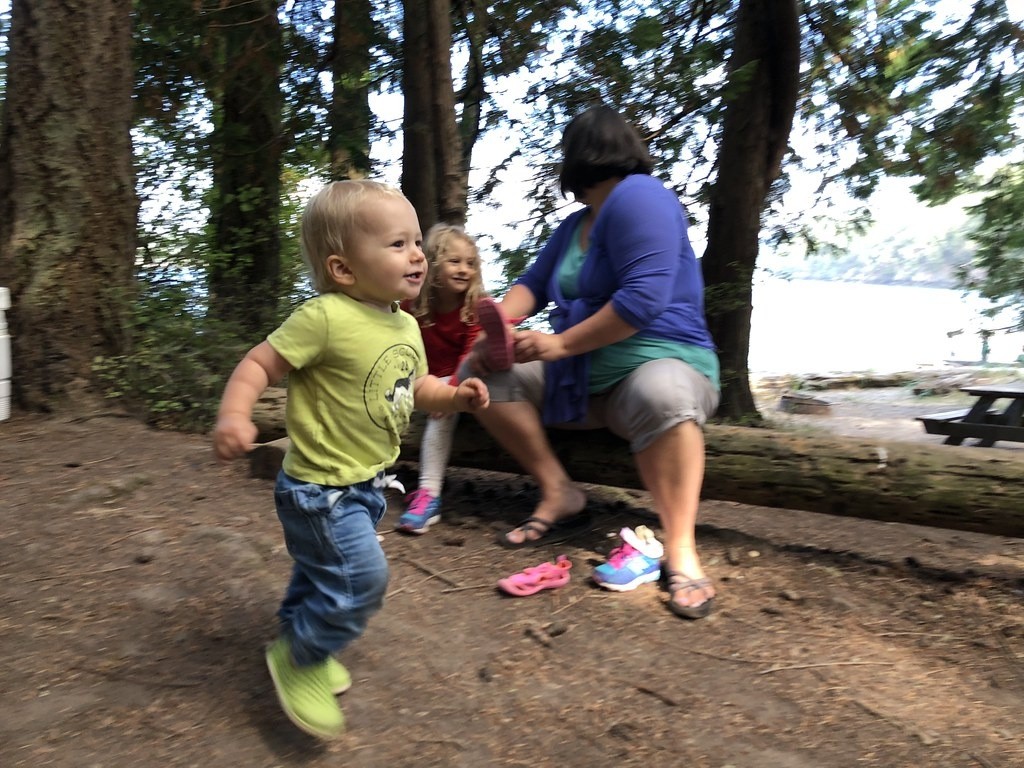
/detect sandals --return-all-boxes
[476,298,528,370]
[498,555,572,596]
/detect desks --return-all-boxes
[922,384,1024,446]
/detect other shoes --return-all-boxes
[265,639,345,741]
[266,632,351,694]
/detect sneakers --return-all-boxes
[592,543,661,592]
[400,489,443,534]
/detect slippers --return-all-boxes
[497,517,595,548]
[662,563,712,617]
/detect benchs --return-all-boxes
[915,408,998,422]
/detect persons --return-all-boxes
[456,106,720,615]
[401,222,512,534]
[215,179,489,739]
